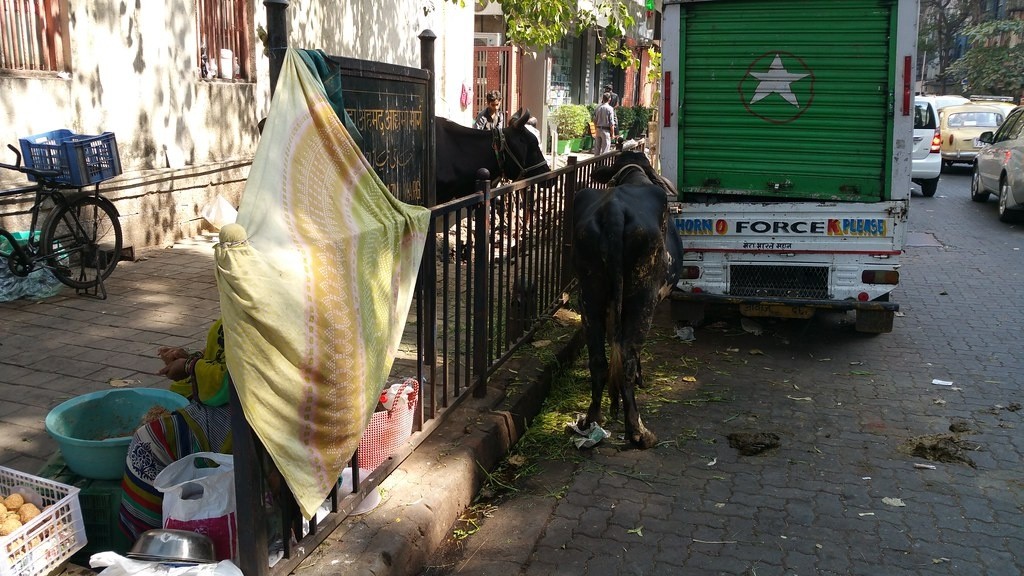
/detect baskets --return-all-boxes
[1,466,86,576]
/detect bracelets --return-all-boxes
[183,355,197,377]
[189,351,205,360]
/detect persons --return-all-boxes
[116,312,253,554]
[592,92,615,170]
[600,84,622,146]
[521,116,542,204]
[472,90,505,130]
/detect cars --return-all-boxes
[939,106,1007,169]
[971,106,1024,222]
[962,95,1020,119]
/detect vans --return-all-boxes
[910,96,942,197]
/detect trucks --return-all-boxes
[657,1,920,336]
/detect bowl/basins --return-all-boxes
[44,387,190,479]
[125,529,219,565]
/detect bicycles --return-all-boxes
[0,144,124,300]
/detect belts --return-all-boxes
[597,126,610,130]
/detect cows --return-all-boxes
[258,107,558,299]
[564,151,684,451]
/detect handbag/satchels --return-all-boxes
[152,451,242,571]
[347,378,418,473]
